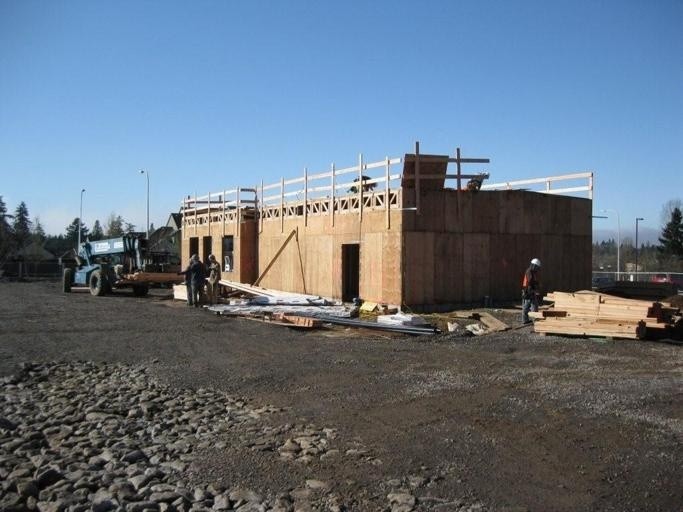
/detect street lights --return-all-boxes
[600,209,620,282]
[139,170,150,240]
[77,189,85,255]
[635,218,643,282]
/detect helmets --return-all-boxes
[531,258,541,266]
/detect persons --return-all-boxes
[351,175,376,192]
[467,180,481,193]
[520,258,542,326]
[177,254,221,308]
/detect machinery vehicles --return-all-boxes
[62,234,151,296]
